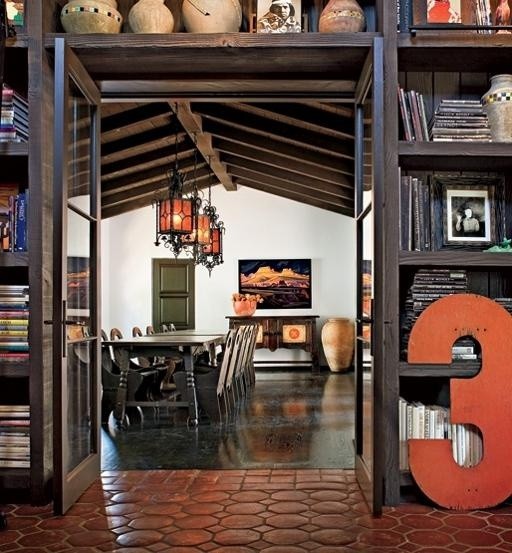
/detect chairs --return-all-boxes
[100,322,258,436]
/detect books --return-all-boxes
[399,267,512,474]
[398,166,432,253]
[0,88,30,469]
[398,0,497,144]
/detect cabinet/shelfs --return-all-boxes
[226,313,320,382]
[0,47,53,507]
[355,45,512,522]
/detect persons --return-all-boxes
[259,0,301,33]
[455,207,480,232]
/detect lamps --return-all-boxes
[150,106,229,276]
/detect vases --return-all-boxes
[233,303,257,317]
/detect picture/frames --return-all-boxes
[237,259,312,310]
[65,257,89,309]
[428,170,507,252]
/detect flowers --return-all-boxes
[231,293,265,304]
